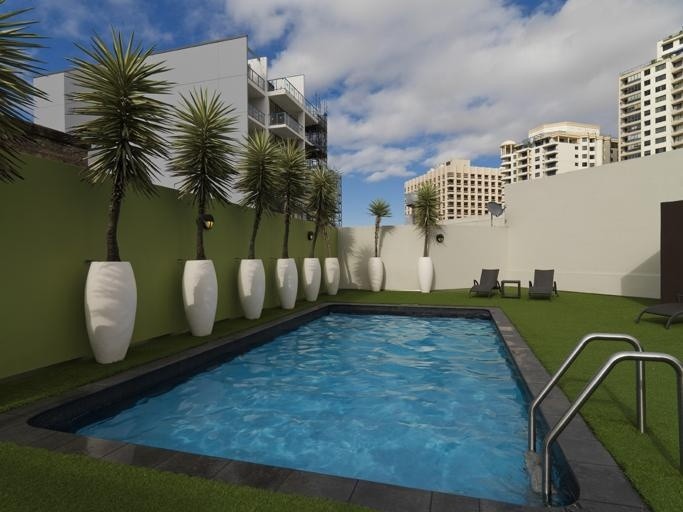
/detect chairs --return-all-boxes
[635,302,682,328]
[468,268,502,298]
[526,269,557,300]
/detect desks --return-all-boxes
[500,280,521,299]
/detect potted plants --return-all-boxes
[365,196,392,291]
[234,129,344,322]
[410,180,441,293]
